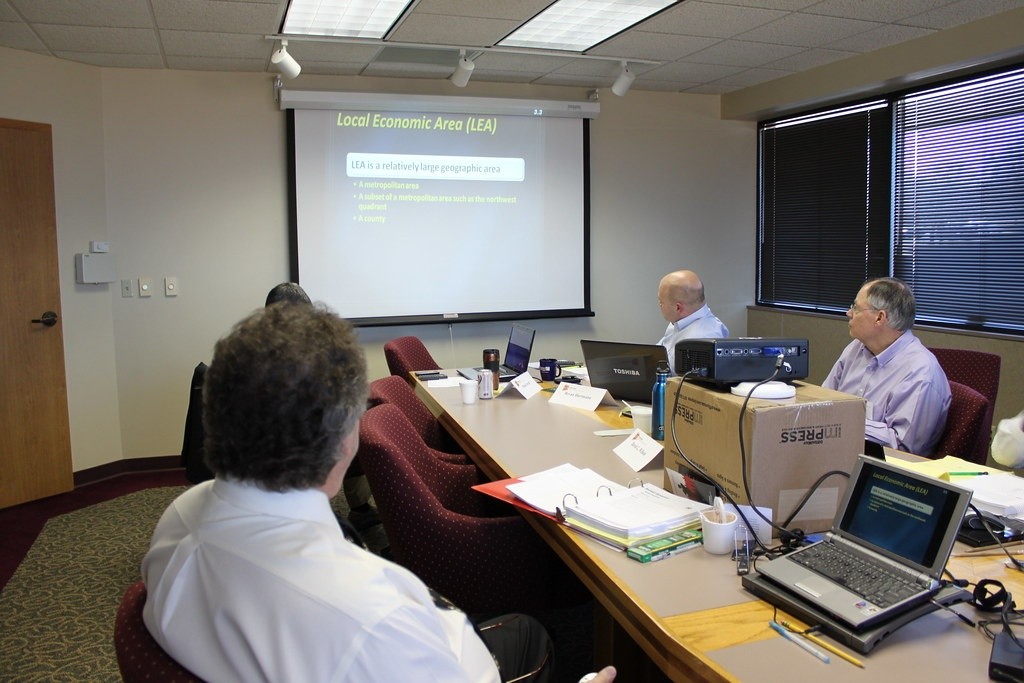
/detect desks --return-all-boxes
[410,362,1024,683]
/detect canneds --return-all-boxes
[477,369,493,399]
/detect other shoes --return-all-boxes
[349,507,383,532]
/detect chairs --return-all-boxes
[352,335,537,604]
[928,343,1001,466]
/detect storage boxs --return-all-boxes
[661,375,864,545]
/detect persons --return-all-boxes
[140,297,617,683]
[820,277,952,458]
[655,269,729,377]
[264,281,377,522]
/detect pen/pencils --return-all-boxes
[950,551,1024,557]
[781,621,865,668]
[965,540,1024,553]
[769,621,830,662]
[949,472,989,475]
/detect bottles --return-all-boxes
[483,349,499,391]
[651,360,671,440]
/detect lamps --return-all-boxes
[449,51,474,89]
[271,40,302,80]
[613,60,637,100]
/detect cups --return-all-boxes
[540,358,561,381]
[631,405,652,433]
[460,380,479,403]
[701,509,738,555]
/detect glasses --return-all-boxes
[850,304,874,315]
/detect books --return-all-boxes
[471,462,715,554]
[951,470,1024,517]
